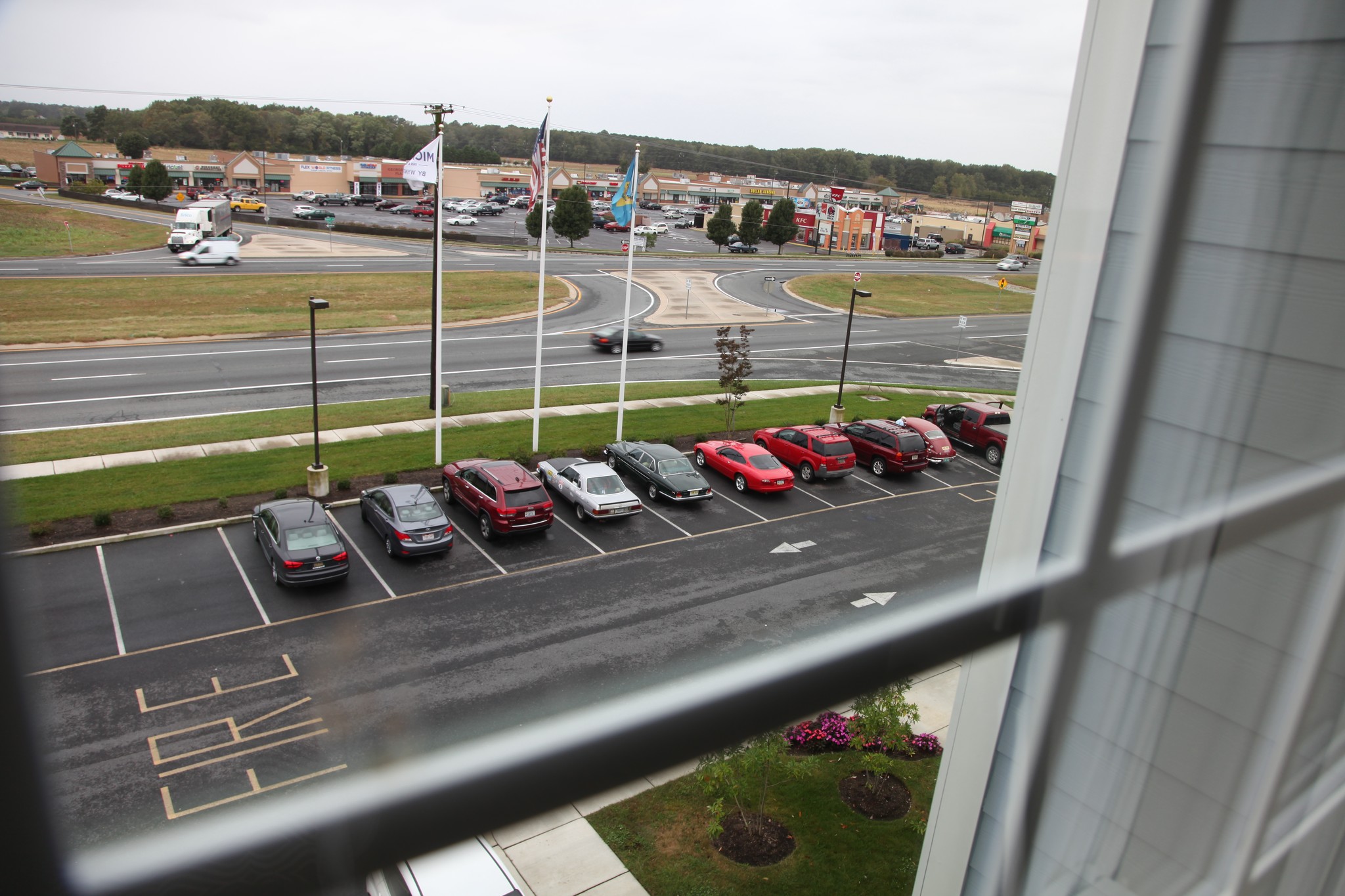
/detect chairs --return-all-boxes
[953,423,961,431]
[660,460,677,468]
[642,458,654,468]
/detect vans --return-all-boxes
[1000,254,1029,268]
[945,243,966,254]
[177,236,242,266]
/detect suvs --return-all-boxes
[908,233,944,250]
[441,457,554,542]
[751,424,857,483]
[821,419,929,478]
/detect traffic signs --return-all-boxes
[764,276,776,282]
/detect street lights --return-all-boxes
[305,295,331,497]
[828,288,872,421]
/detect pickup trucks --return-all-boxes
[920,401,1011,466]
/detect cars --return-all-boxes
[0,164,711,238]
[728,242,758,253]
[603,440,714,505]
[693,440,795,496]
[877,417,957,465]
[722,235,742,246]
[359,483,454,559]
[885,214,917,224]
[996,258,1023,271]
[251,496,350,591]
[535,457,643,522]
[587,325,665,356]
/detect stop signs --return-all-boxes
[853,272,861,282]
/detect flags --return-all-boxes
[527,113,548,215]
[403,136,439,191]
[610,155,638,227]
[901,198,918,206]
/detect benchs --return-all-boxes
[399,506,437,518]
[853,251,861,257]
[846,251,854,257]
[287,529,330,541]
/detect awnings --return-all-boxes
[381,178,432,184]
[581,186,587,190]
[265,175,291,180]
[359,177,379,182]
[607,187,620,192]
[119,170,131,176]
[94,168,117,175]
[660,189,883,206]
[587,186,607,191]
[481,182,543,189]
[992,226,1013,238]
[193,172,225,178]
[168,171,190,177]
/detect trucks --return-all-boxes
[166,199,233,253]
